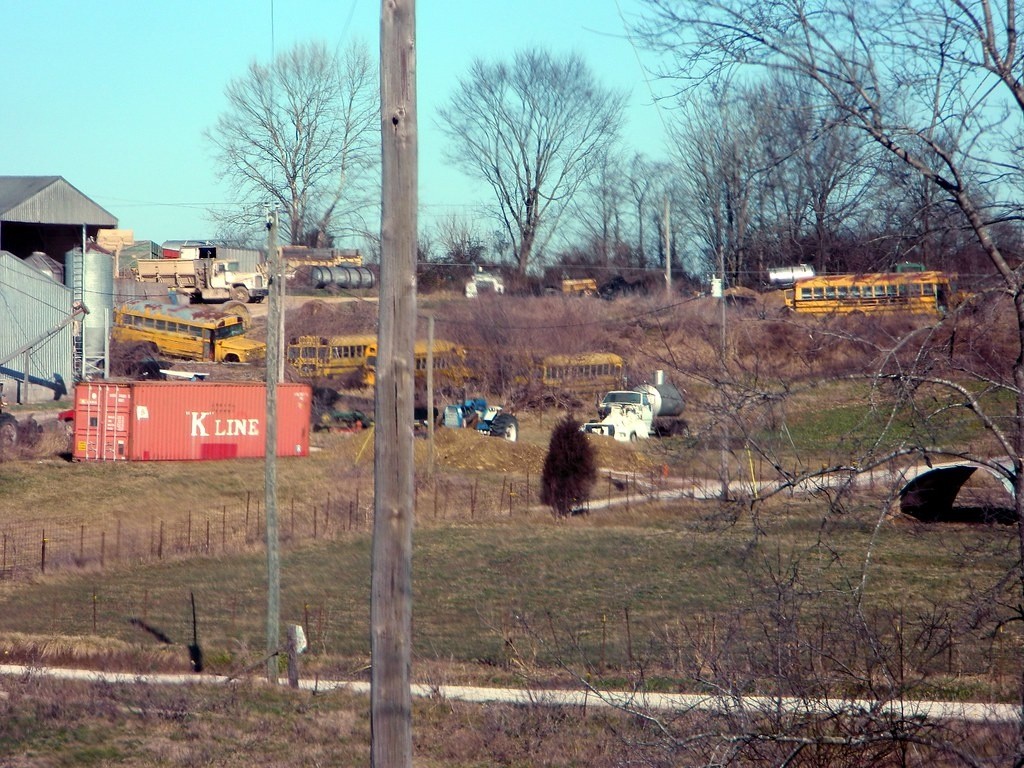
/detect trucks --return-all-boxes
[131,256,271,305]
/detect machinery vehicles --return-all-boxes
[577,381,691,442]
[440,393,519,446]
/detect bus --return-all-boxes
[562,276,598,299]
[362,340,470,387]
[110,298,268,367]
[508,350,625,402]
[286,333,378,381]
[783,271,980,321]
